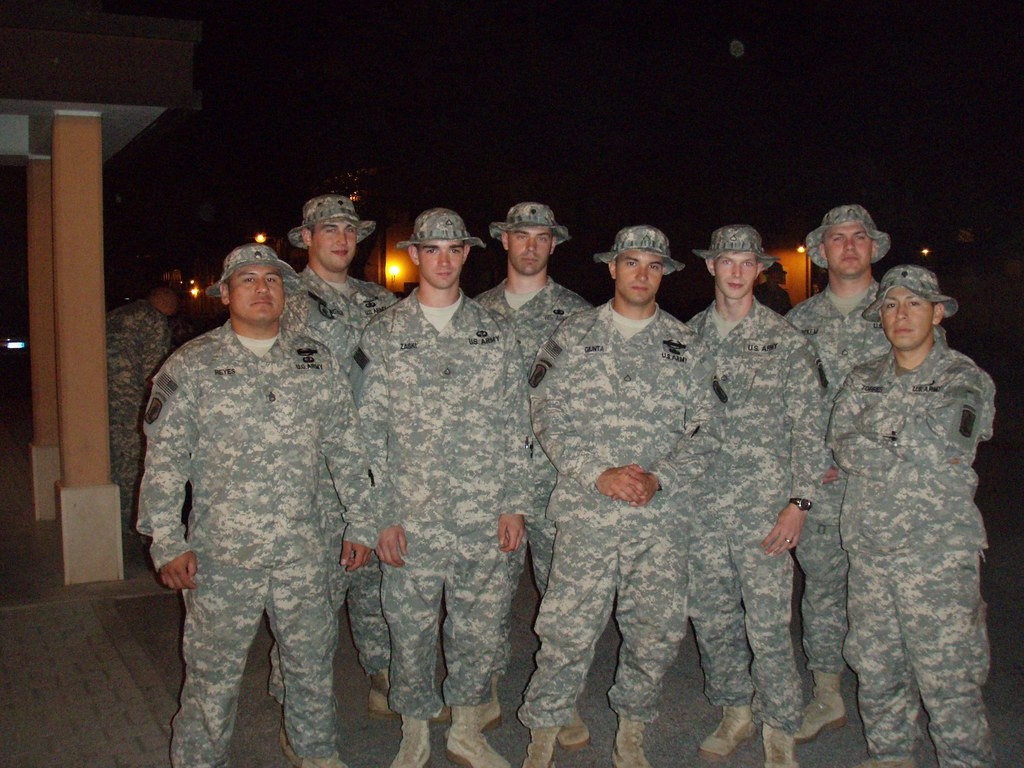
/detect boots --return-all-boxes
[445,673,502,740]
[557,717,591,750]
[368,667,450,723]
[448,705,512,768]
[698,705,757,762]
[390,715,432,768]
[794,671,847,743]
[521,726,563,768]
[611,716,651,768]
[761,722,799,768]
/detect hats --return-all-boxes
[205,243,300,299]
[806,205,891,269]
[397,208,487,248]
[489,202,570,245]
[692,224,780,271]
[288,194,376,249]
[862,264,959,322]
[593,224,686,275]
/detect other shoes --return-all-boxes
[278,722,348,768]
[857,759,917,768]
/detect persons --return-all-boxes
[106,283,181,551]
[780,205,948,749]
[138,242,379,768]
[752,261,828,316]
[681,224,829,768]
[825,266,1003,768]
[516,225,726,768]
[270,192,596,768]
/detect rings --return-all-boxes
[785,538,791,544]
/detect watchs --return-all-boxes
[789,498,813,511]
